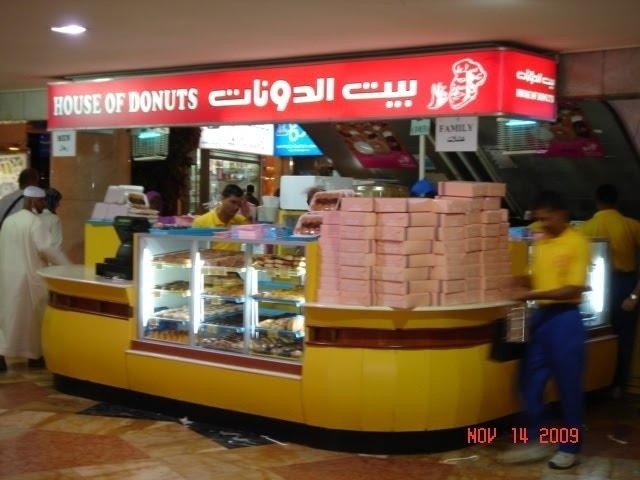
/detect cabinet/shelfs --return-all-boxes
[134,149,266,225]
[134,231,316,364]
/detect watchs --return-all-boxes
[628,293,639,302]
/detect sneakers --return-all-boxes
[547,452,578,471]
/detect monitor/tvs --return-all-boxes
[113,216,150,244]
[274,121,325,157]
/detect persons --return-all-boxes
[244,182,258,206]
[1,184,74,377]
[620,285,639,313]
[491,196,595,471]
[37,185,64,269]
[1,166,39,222]
[188,184,252,255]
[576,186,639,401]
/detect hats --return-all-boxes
[22,185,47,199]
